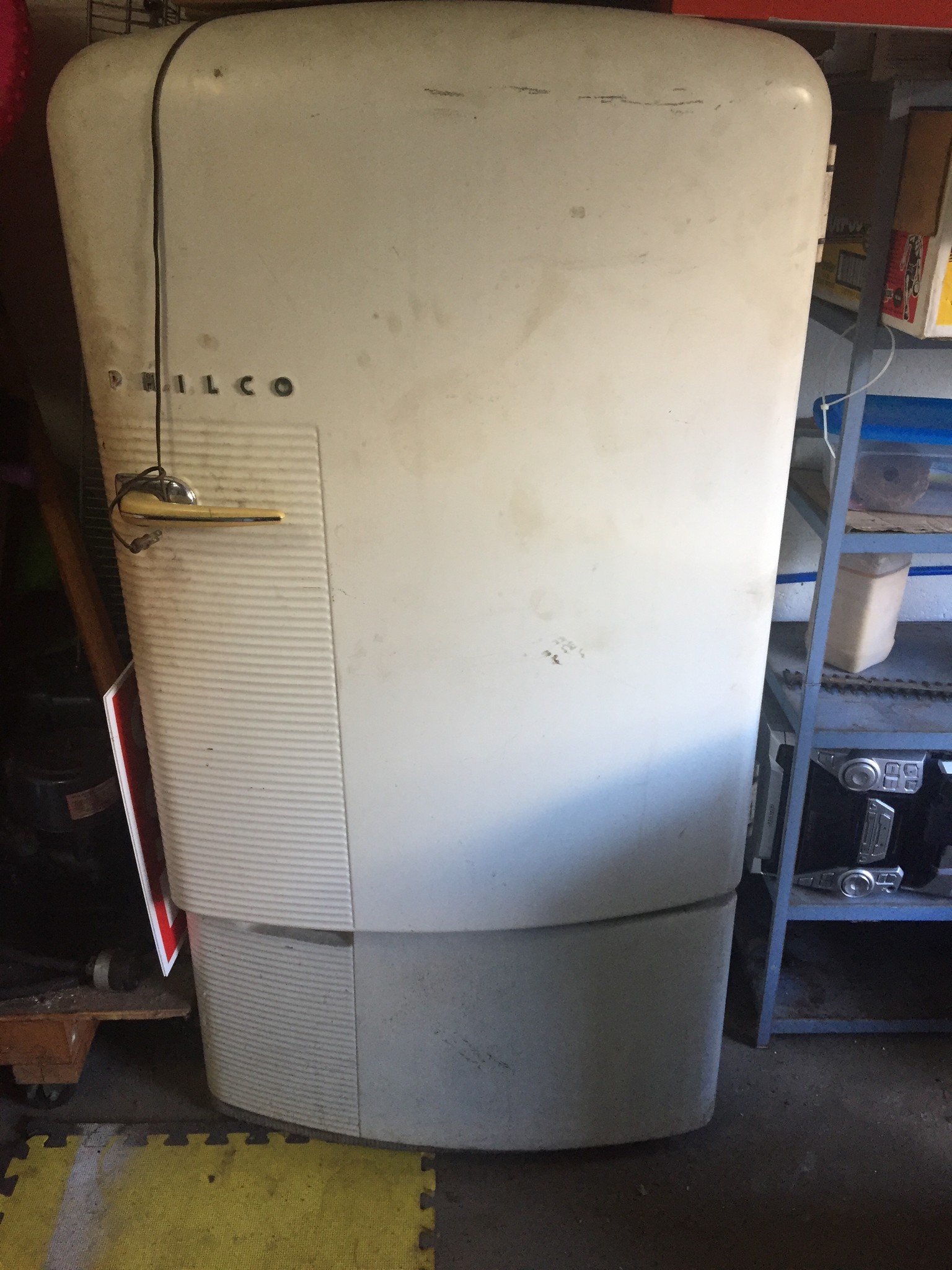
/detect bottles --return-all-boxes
[808,542,914,674]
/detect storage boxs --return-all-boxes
[809,386,952,518]
[814,102,952,342]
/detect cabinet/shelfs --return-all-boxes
[739,78,952,1052]
[0,390,195,1023]
